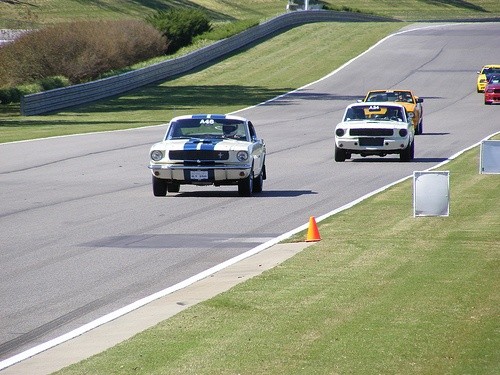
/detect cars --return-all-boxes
[475,65,500,93]
[144,112,268,197]
[484,74,500,105]
[334,101,416,162]
[356,89,424,135]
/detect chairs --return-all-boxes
[353,109,364,118]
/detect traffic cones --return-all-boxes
[305,216,322,242]
